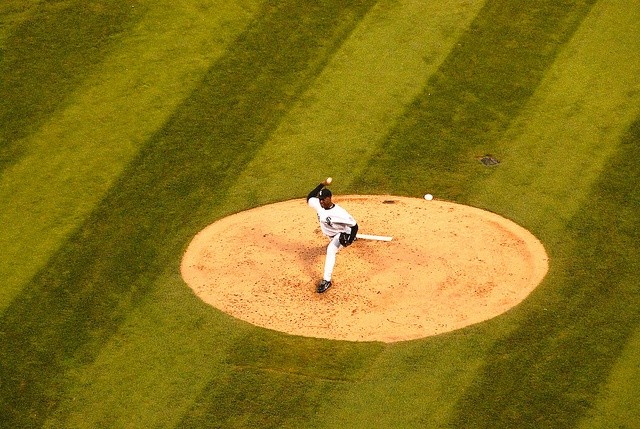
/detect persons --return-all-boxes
[307,177,358,293]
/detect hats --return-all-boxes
[319,189,331,200]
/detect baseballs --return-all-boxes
[424,194,433,200]
[327,177,332,184]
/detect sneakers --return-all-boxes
[317,280,332,293]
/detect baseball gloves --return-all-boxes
[340,233,351,247]
